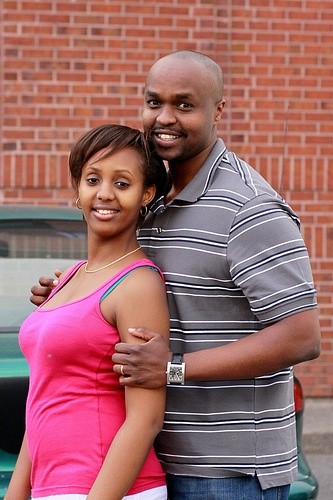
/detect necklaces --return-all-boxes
[84,245,142,275]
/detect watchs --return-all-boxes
[166,353,188,388]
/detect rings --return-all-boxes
[121,365,126,375]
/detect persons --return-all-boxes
[4,124,170,500]
[29,49,322,500]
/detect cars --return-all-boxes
[0,198,318,499]
